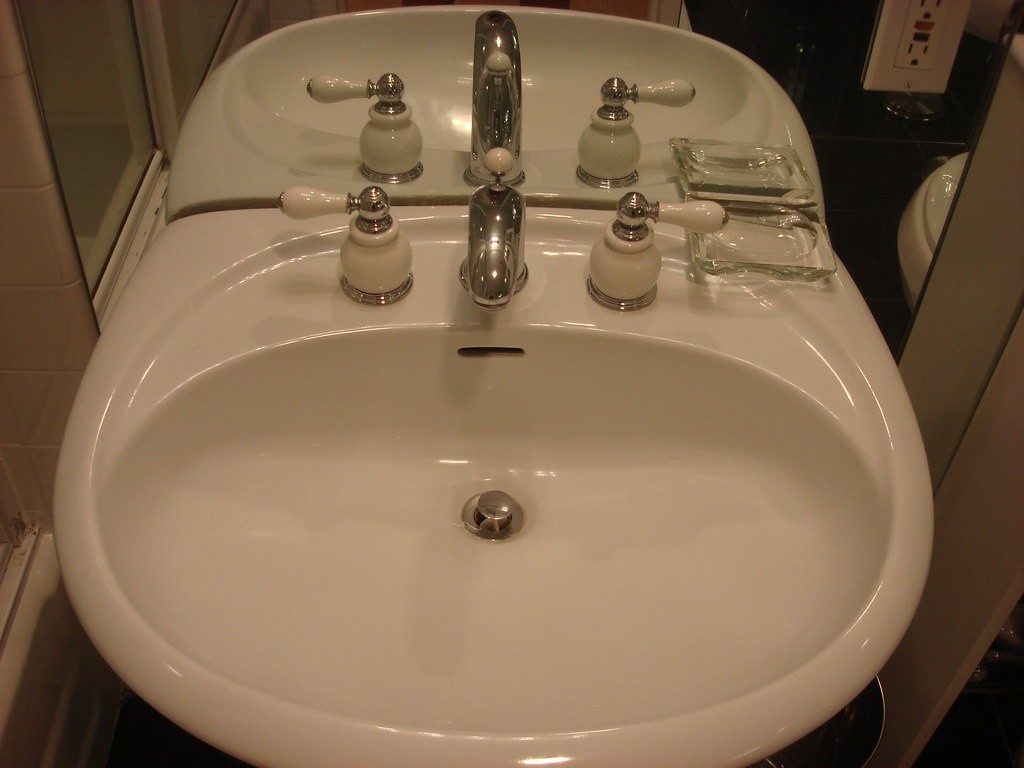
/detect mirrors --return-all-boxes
[0,0,1024,367]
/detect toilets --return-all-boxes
[893,148,974,314]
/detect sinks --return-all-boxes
[49,207,937,768]
[162,5,827,227]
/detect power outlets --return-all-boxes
[862,0,971,94]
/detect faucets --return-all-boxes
[461,9,527,188]
[458,145,530,316]
